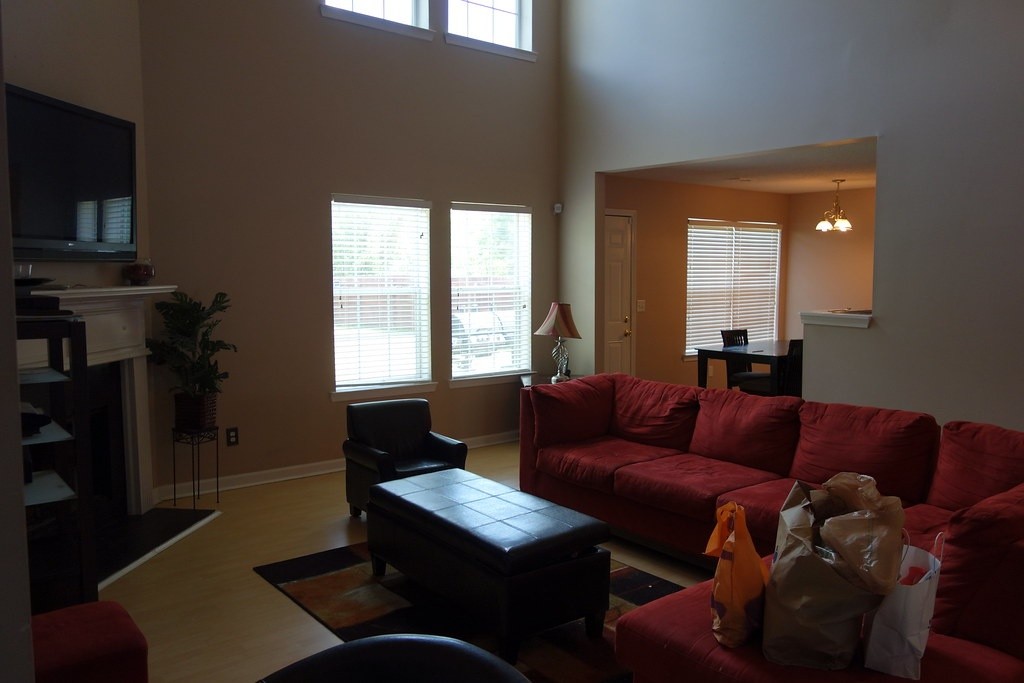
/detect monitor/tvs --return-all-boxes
[4,81,137,263]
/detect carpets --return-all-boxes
[254,540,685,683]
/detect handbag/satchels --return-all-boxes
[761,471,907,672]
[864,529,945,680]
[694,494,767,652]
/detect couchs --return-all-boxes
[340,398,468,518]
[520,375,1023,683]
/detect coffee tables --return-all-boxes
[369,467,610,666]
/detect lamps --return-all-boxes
[533,302,581,376]
[816,179,853,232]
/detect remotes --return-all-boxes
[14,277,56,287]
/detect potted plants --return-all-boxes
[146,290,239,429]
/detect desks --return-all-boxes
[695,341,791,395]
[172,426,221,510]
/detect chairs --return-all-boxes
[720,330,771,390]
[775,339,803,397]
